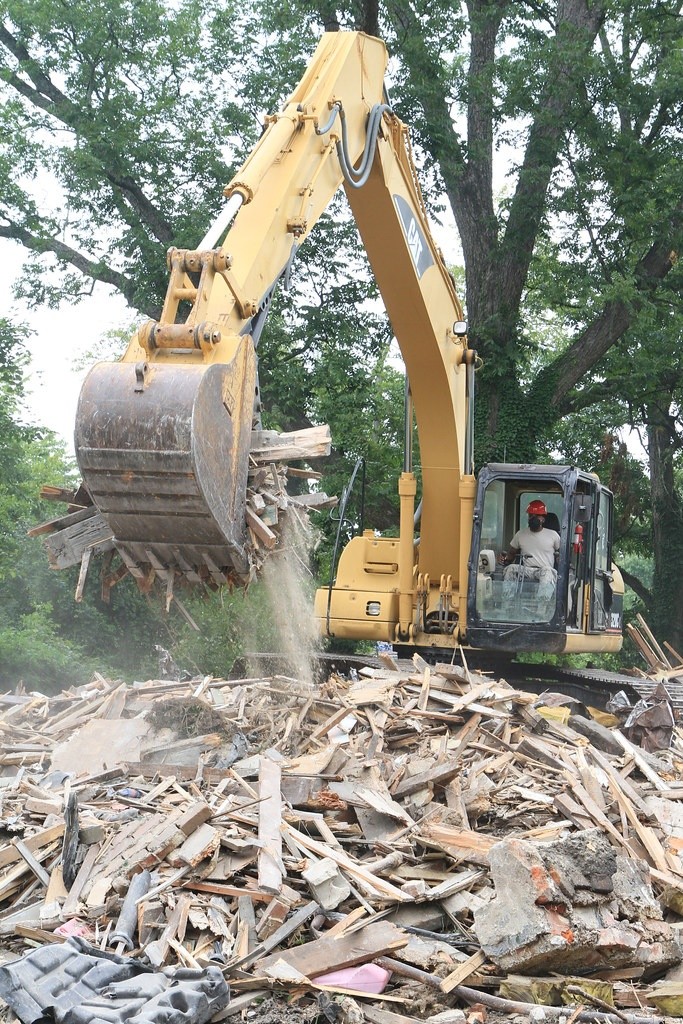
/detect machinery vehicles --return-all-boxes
[74,29,645,750]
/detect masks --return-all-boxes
[528,516,544,532]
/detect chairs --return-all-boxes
[512,511,560,593]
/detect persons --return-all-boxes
[497,500,562,619]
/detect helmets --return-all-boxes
[525,500,547,515]
